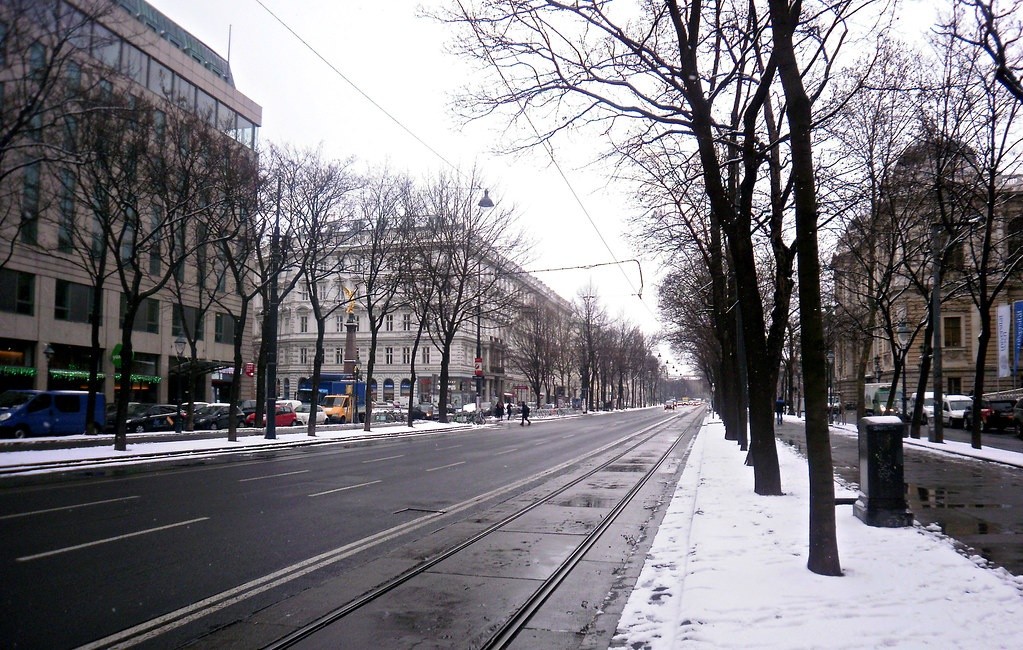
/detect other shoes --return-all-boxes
[528,422,531,426]
[520,422,524,426]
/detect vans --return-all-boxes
[0,389,108,440]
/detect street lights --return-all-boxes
[897,320,912,423]
[826,350,836,425]
[173,329,188,435]
[352,356,363,424]
[928,224,952,445]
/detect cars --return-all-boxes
[663,397,706,411]
[845,401,857,411]
[504,396,646,418]
[106,389,493,433]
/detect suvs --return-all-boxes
[963,397,1018,434]
[906,390,945,424]
[1010,397,1023,439]
[941,395,973,430]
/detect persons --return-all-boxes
[775,397,786,425]
[507,403,513,420]
[495,400,506,420]
[519,401,531,426]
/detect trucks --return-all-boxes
[864,381,920,420]
[827,395,843,415]
